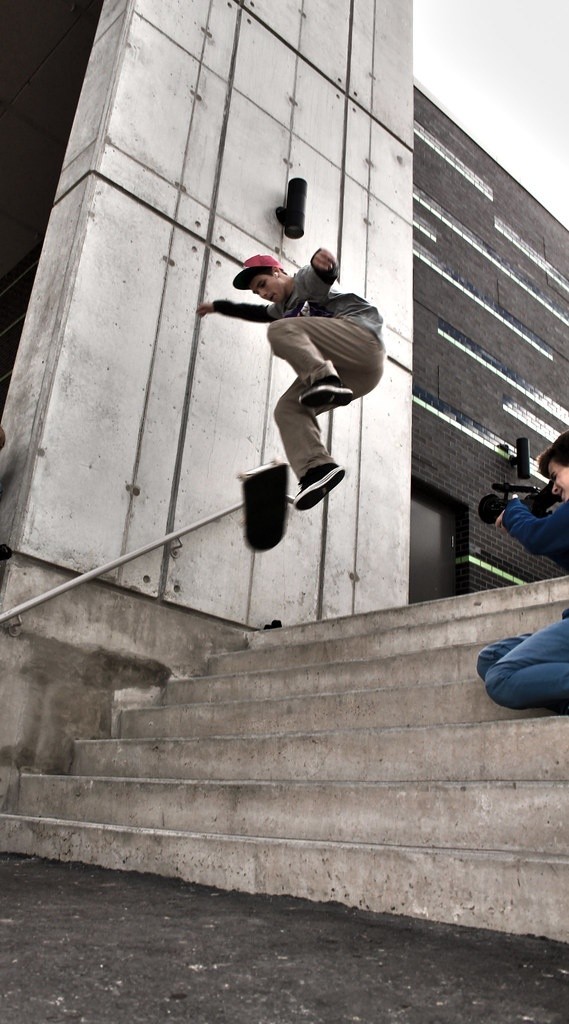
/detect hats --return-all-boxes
[231,254,285,290]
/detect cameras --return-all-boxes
[478,479,562,526]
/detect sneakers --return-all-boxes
[294,374,354,407]
[291,461,346,510]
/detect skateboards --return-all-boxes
[238,460,288,552]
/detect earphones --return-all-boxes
[274,272,279,279]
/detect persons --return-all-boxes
[476,431,569,717]
[197,248,385,511]
